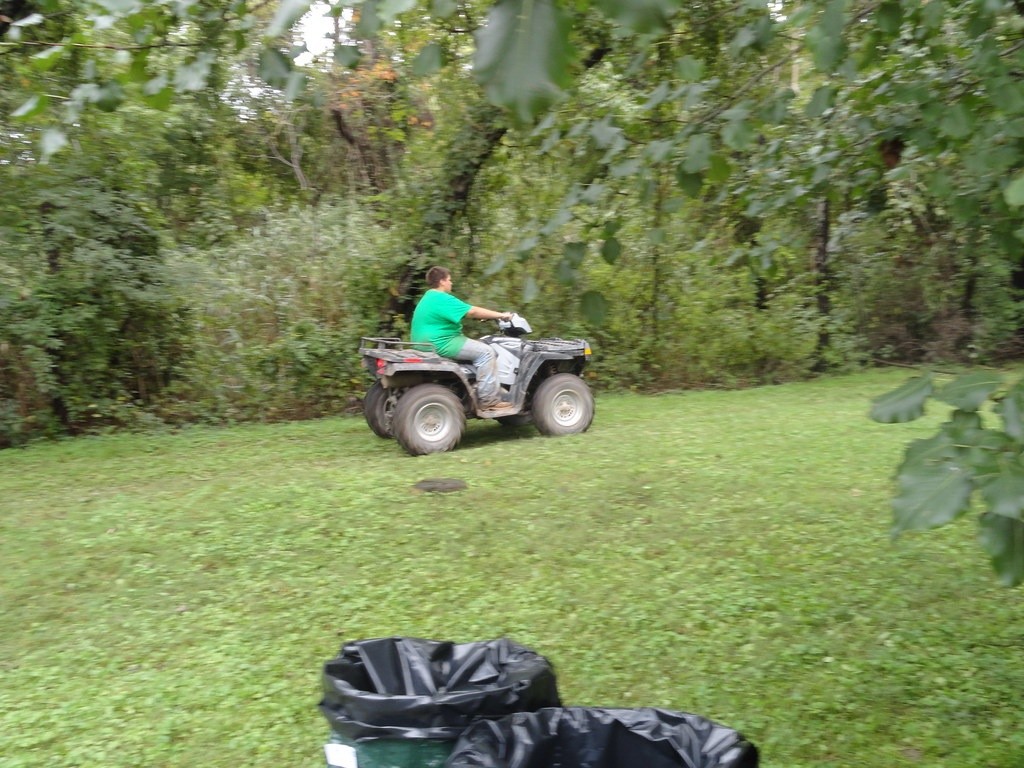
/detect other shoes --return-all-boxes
[480,401,514,410]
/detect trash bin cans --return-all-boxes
[319,634,558,768]
[436,705,760,768]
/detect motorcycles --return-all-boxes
[353,312,600,456]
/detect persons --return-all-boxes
[410,266,513,410]
